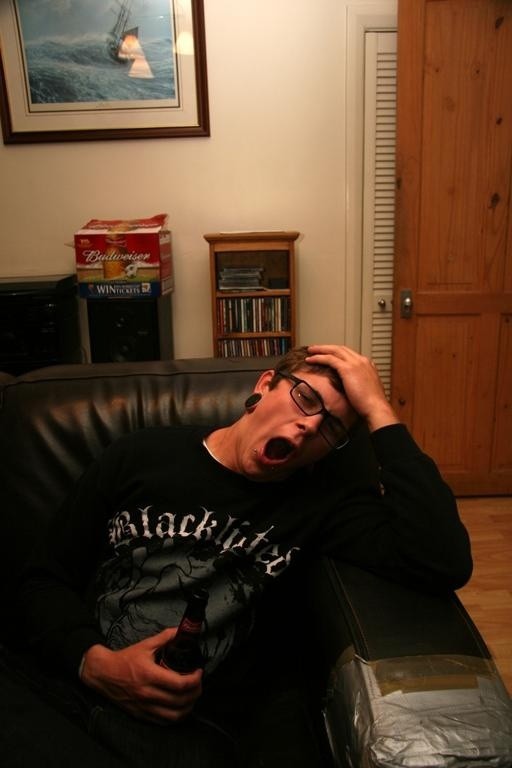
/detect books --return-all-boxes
[216,265,289,356]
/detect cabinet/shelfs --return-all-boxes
[203,231,300,358]
[0,273,82,376]
[87,294,173,364]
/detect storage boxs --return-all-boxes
[64,214,174,298]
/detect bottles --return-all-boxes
[155,585,211,673]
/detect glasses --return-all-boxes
[279,369,353,452]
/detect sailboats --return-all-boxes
[106,0,139,65]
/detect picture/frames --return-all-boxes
[0,0,210,144]
